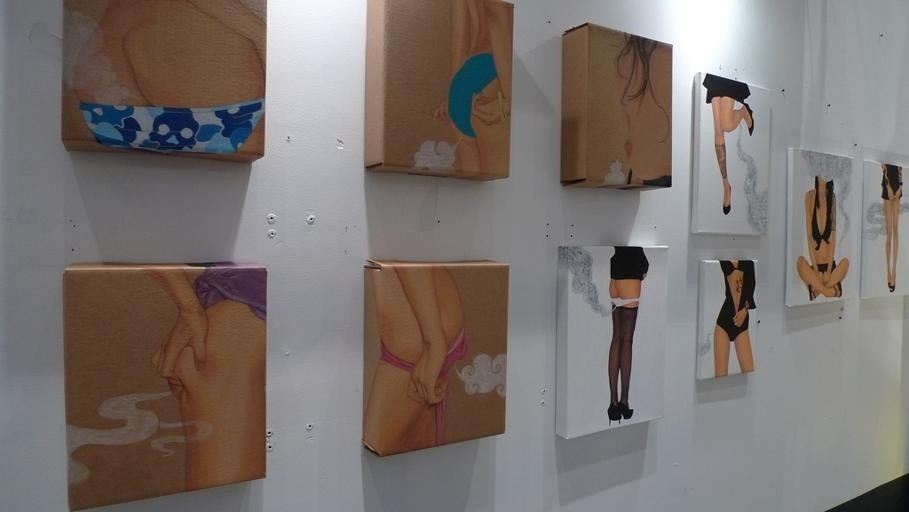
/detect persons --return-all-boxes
[362,268,466,457]
[796,176,850,303]
[154,267,268,492]
[714,260,758,378]
[622,36,673,186]
[432,1,511,180]
[608,246,650,426]
[703,73,754,217]
[73,1,263,154]
[880,166,903,294]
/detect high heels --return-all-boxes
[608,402,633,426]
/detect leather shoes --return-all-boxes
[723,204,732,215]
[743,103,754,136]
[888,281,896,293]
